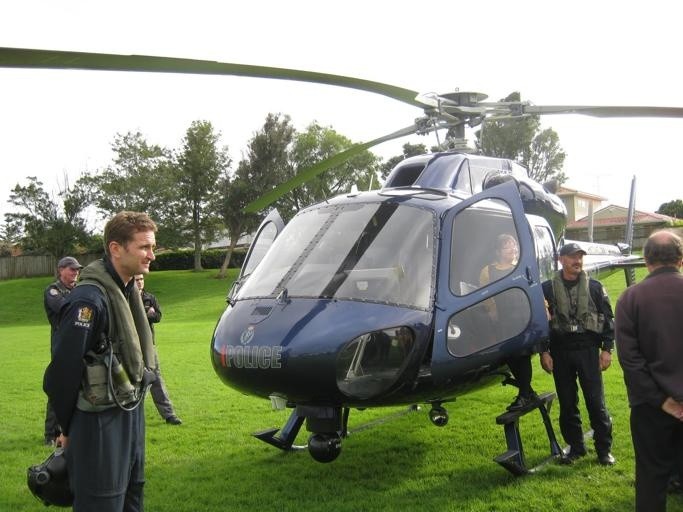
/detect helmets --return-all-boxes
[27,448,74,507]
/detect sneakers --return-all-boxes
[560,440,586,464]
[598,448,614,464]
[166,416,181,424]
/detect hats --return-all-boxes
[58,256,83,269]
[561,243,587,255]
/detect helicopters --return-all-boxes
[0,47,682,478]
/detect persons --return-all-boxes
[476,231,540,411]
[540,242,616,467]
[615,229,683,512]
[42,209,161,511]
[42,255,81,446]
[133,274,183,426]
